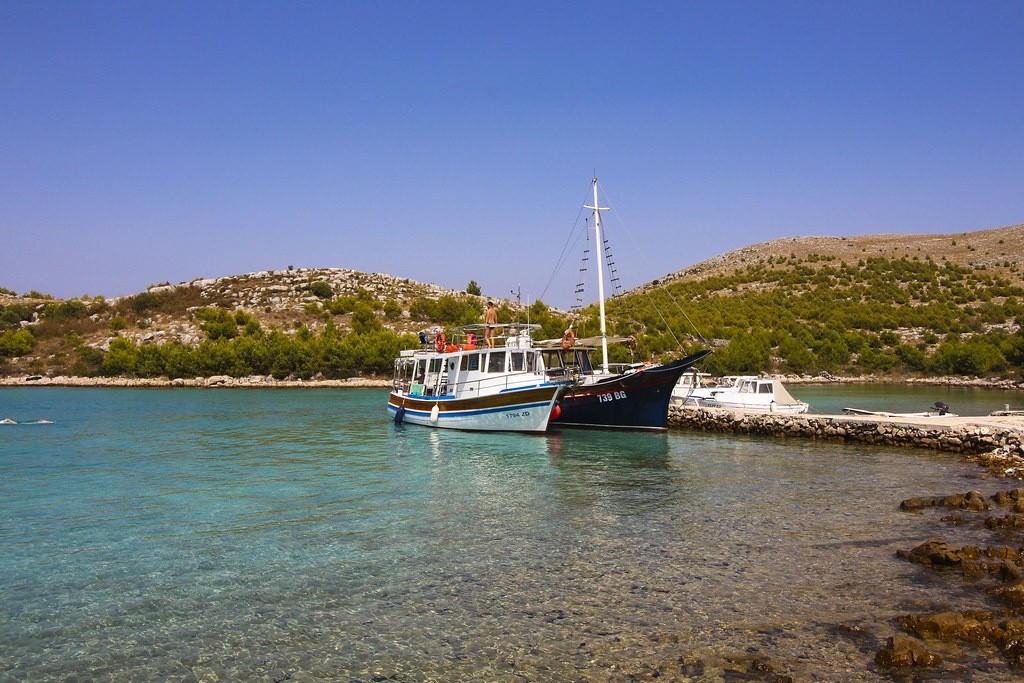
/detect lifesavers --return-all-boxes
[626,335,638,350]
[436,333,446,352]
[562,330,575,350]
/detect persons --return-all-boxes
[414,368,425,384]
[934,402,950,416]
[484,302,497,349]
[711,377,722,387]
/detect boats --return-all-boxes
[384,320,568,436]
[533,341,810,415]
[842,400,958,419]
[508,170,715,435]
